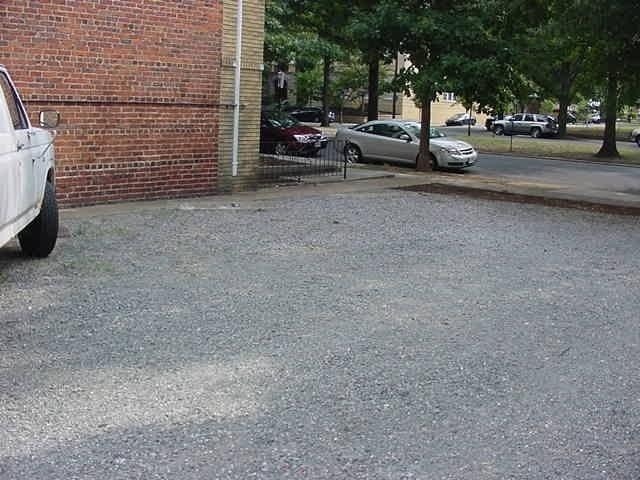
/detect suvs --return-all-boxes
[0,64,59,261]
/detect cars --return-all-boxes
[284,105,336,125]
[631,128,640,148]
[261,109,330,158]
[446,112,477,127]
[484,102,602,138]
[334,119,478,172]
